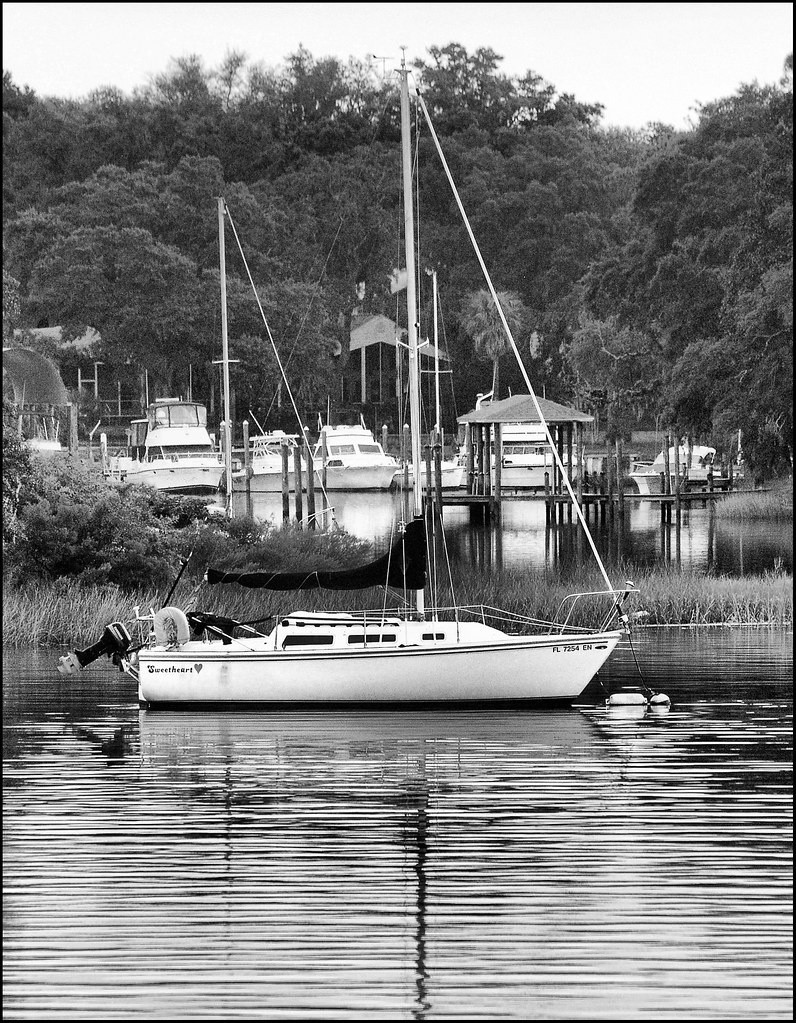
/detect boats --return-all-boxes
[232,394,403,492]
[102,360,224,494]
[629,445,743,494]
[456,441,587,491]
[390,454,466,489]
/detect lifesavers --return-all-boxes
[153,606,190,647]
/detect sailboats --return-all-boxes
[55,45,653,709]
